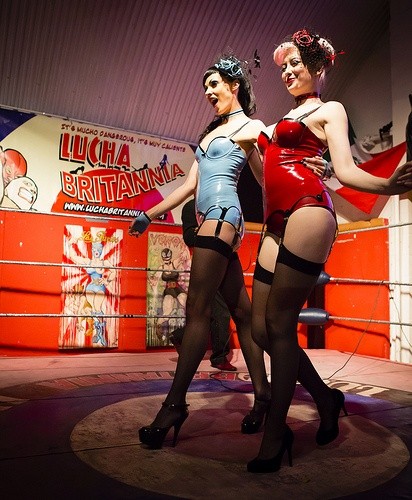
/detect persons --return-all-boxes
[247,38,411,473]
[168,195,237,371]
[128,56,333,449]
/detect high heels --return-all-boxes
[138,401,189,451]
[241,398,273,433]
[316,389,349,443]
[244,422,296,476]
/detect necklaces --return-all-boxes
[295,92,322,104]
[220,109,244,121]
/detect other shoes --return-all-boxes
[167,334,179,354]
[211,356,236,371]
